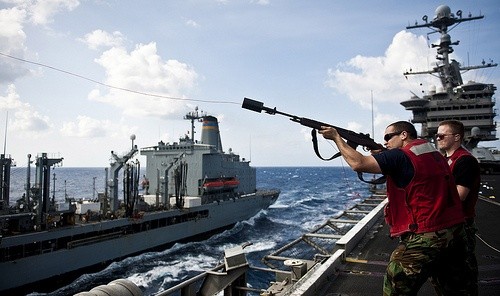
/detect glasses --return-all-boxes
[384,131,402,142]
[434,133,455,140]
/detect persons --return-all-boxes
[435,120,482,296]
[317,121,461,296]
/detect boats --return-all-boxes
[0,99,281,296]
[202,180,240,194]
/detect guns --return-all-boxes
[241,97,387,153]
